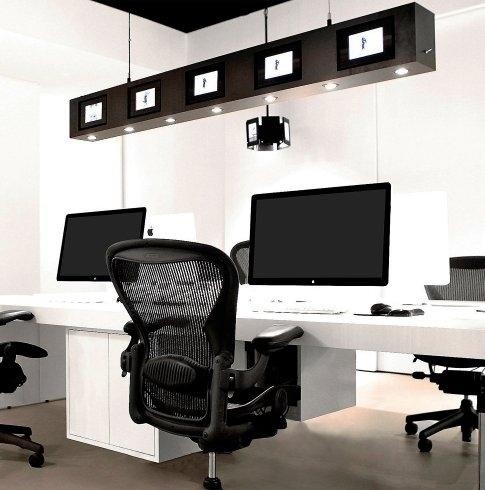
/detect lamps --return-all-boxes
[246,8,291,152]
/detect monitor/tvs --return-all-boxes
[55,207,146,282]
[385,191,450,286]
[247,182,391,286]
[142,213,195,242]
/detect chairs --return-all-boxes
[405,256,485,453]
[0,310,49,468]
[106,238,304,490]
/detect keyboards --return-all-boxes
[251,305,348,316]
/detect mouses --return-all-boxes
[410,309,424,316]
[386,309,411,317]
[369,303,391,315]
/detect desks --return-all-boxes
[0,292,485,465]
